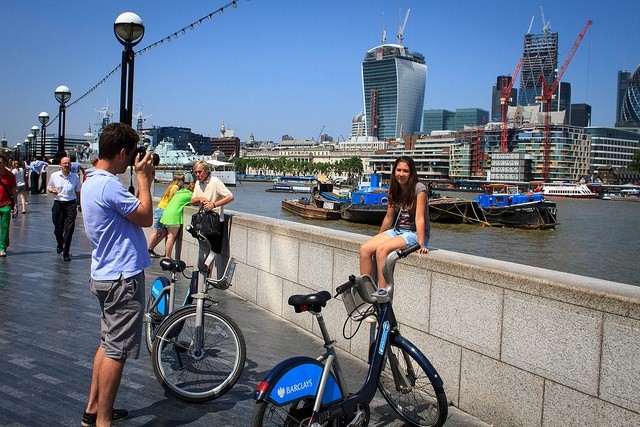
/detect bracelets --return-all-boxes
[213,203,216,208]
[420,244,426,248]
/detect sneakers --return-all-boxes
[366,312,377,323]
[0,251,6,256]
[148,248,160,259]
[80,407,128,426]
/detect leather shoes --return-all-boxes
[57,244,63,254]
[64,256,71,261]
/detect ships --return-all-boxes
[533,183,596,199]
[596,182,640,203]
[341,169,477,222]
[309,183,352,204]
[154,160,237,187]
[265,176,333,194]
[473,184,561,230]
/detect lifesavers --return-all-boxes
[381,197,388,205]
[360,195,364,204]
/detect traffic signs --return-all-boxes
[31,126,39,160]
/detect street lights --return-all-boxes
[28,133,35,164]
[52,85,71,166]
[114,12,144,129]
[23,139,31,162]
[14,145,18,162]
[39,112,49,158]
[17,143,21,162]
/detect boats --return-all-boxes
[282,195,341,220]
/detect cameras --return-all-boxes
[129,147,159,166]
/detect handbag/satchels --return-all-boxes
[190,204,223,254]
[1,181,15,208]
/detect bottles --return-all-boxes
[11,210,18,222]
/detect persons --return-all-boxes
[24,160,30,193]
[80,122,155,426]
[39,157,48,194]
[159,181,195,260]
[90,157,98,166]
[47,157,82,262]
[11,161,27,213]
[359,156,431,323]
[190,161,235,289]
[0,155,18,257]
[30,156,45,194]
[70,155,86,212]
[148,174,185,257]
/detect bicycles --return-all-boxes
[251,242,448,427]
[144,224,247,404]
